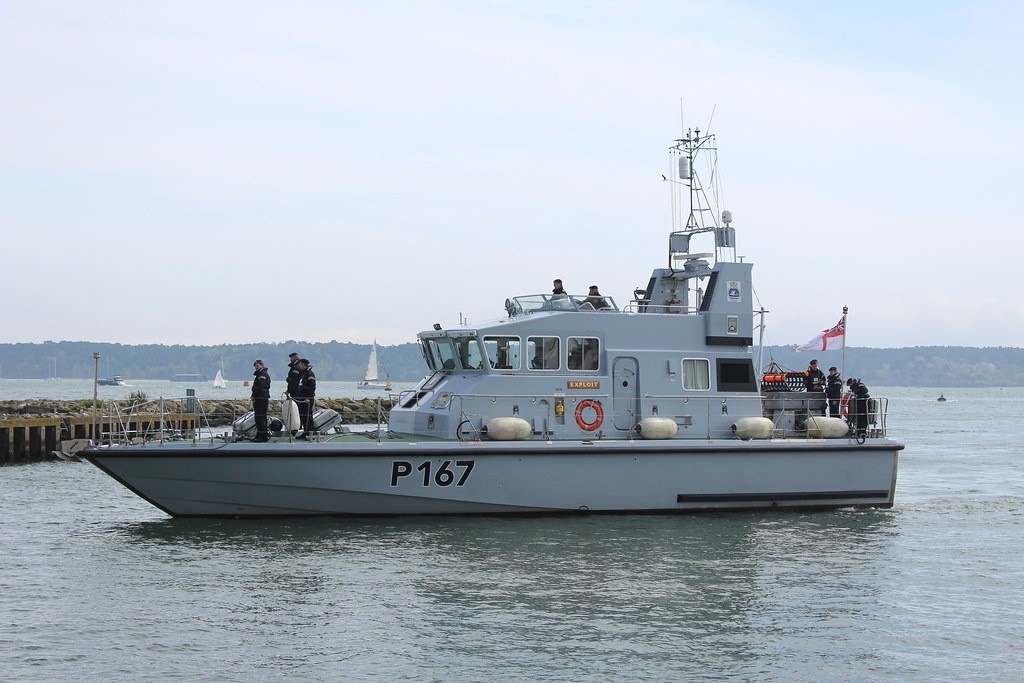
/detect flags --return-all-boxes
[795,316,844,352]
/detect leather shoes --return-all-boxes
[295,432,309,440]
[313,430,319,440]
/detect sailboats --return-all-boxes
[213,369,226,389]
[357,339,392,391]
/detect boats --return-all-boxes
[243,381,249,386]
[937,394,947,401]
[168,374,209,382]
[50,98,905,518]
[97,375,126,385]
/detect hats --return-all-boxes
[289,353,299,357]
[254,360,262,366]
[589,286,597,289]
[300,359,309,364]
[829,367,836,371]
[810,359,818,364]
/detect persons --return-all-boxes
[802,360,826,392]
[286,352,301,399]
[577,286,607,309]
[250,360,270,442]
[846,378,870,437]
[826,367,841,418]
[295,359,316,439]
[551,279,567,295]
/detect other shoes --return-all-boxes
[250,436,268,442]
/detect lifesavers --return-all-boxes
[576,400,603,431]
[842,393,852,418]
